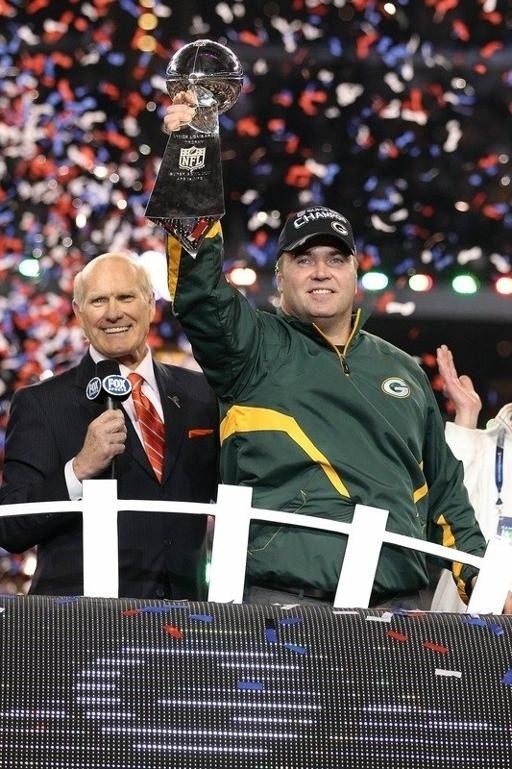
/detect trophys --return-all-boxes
[142,39,244,259]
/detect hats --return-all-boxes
[278,206,355,256]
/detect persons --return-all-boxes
[0,89,512,615]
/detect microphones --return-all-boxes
[85,360,132,462]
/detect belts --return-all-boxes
[251,578,418,608]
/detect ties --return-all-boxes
[128,374,168,485]
